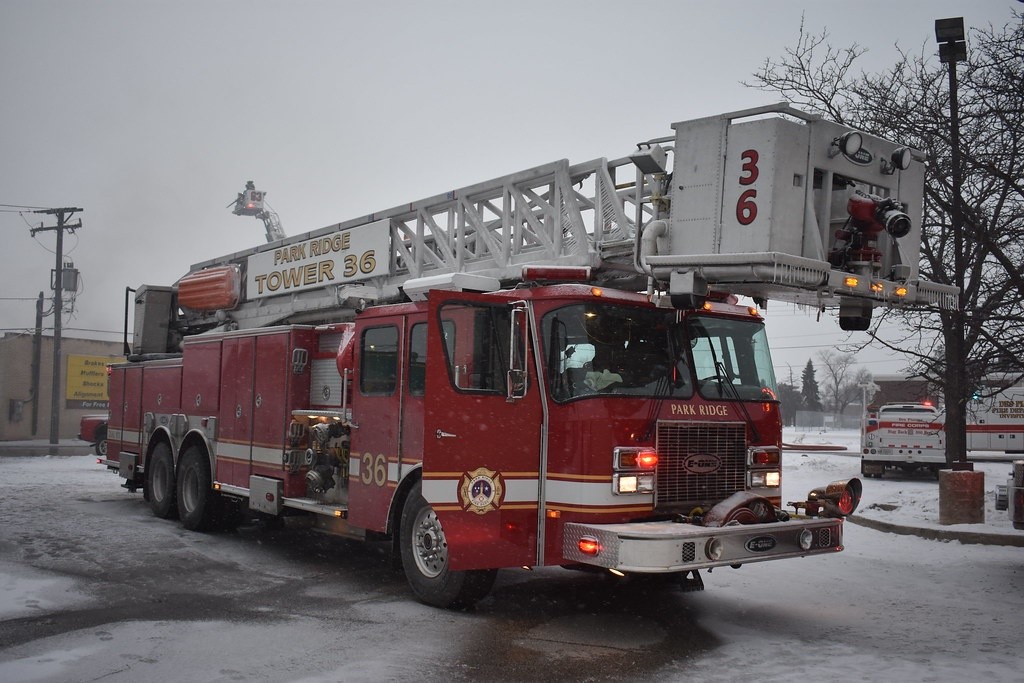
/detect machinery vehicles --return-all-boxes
[859,400,946,478]
[966,371,1024,455]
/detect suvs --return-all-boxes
[78,413,108,455]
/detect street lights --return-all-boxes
[935,17,974,472]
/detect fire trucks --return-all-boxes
[97,103,961,612]
